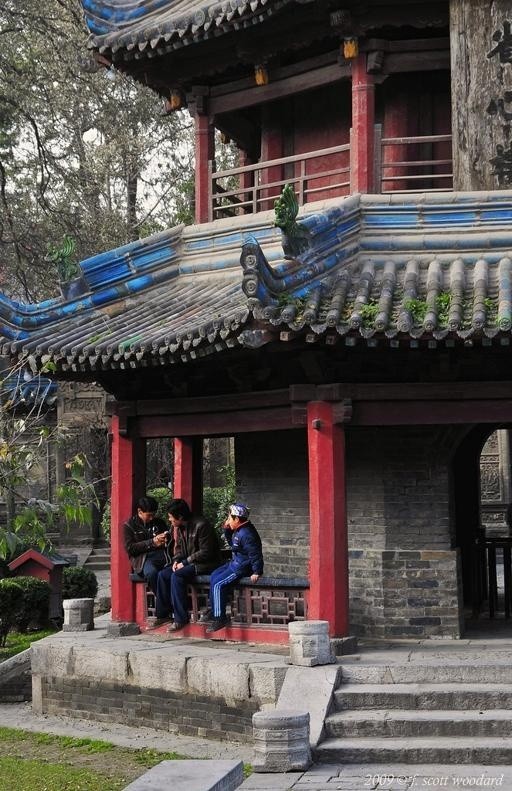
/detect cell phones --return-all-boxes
[163,530,169,535]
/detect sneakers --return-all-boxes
[146,618,174,631]
[206,618,231,633]
[197,611,214,622]
[166,620,189,633]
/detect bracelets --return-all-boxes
[181,557,188,566]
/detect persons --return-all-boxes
[120,496,172,598]
[198,504,264,632]
[145,498,223,633]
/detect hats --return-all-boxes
[229,504,252,518]
